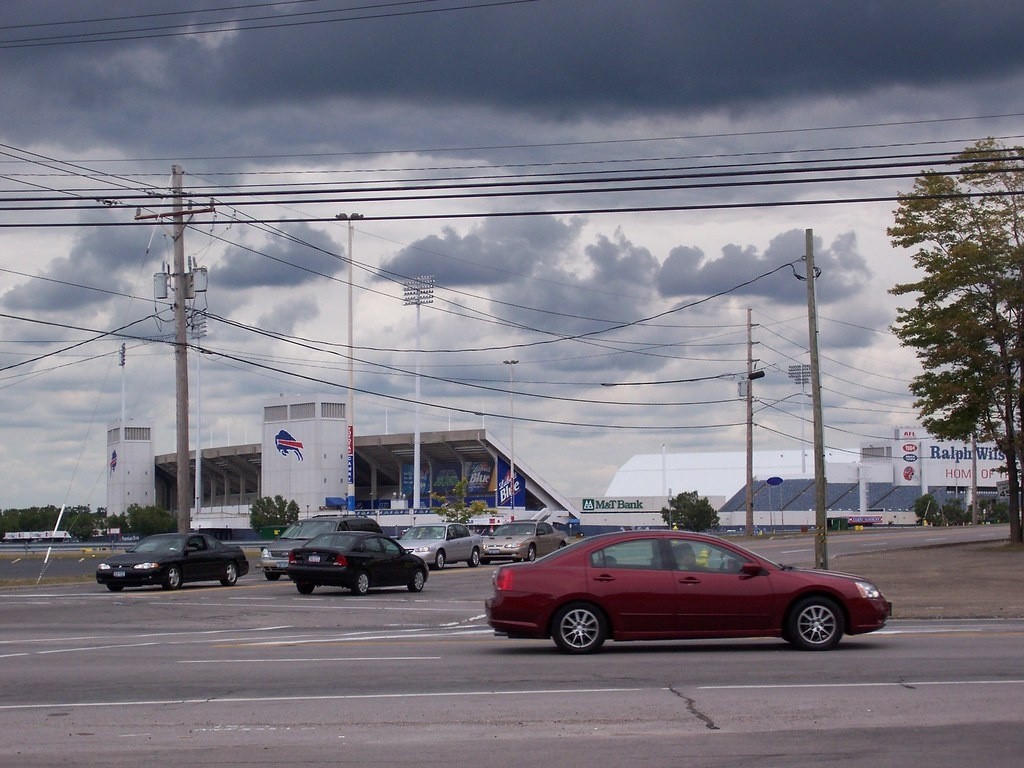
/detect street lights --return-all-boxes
[336,212,366,516]
[402,274,435,509]
[502,360,519,522]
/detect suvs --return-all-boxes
[260,514,383,580]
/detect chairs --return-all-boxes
[421,528,435,539]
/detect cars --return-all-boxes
[479,518,570,566]
[387,522,484,571]
[96,532,249,592]
[485,530,892,655]
[286,530,429,596]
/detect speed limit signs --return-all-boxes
[996,481,1012,499]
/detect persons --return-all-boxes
[674,542,698,571]
